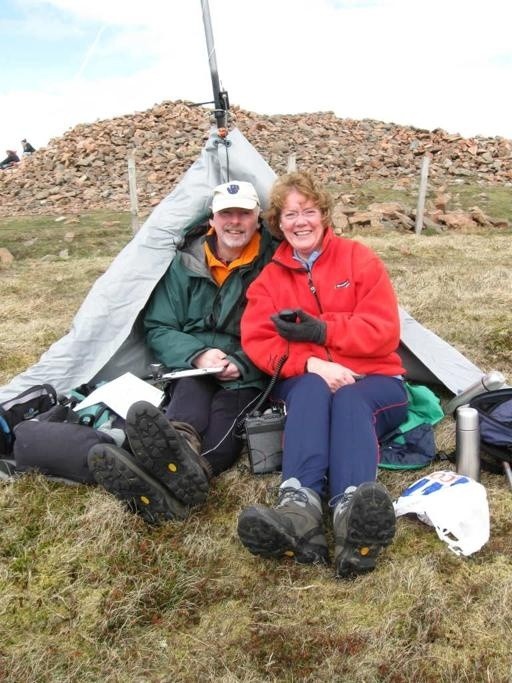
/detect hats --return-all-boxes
[212,179,260,215]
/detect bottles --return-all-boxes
[455,406,481,482]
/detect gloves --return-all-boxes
[269,307,328,346]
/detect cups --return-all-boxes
[277,309,298,323]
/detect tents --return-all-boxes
[1,0,512,404]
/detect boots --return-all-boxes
[334,481,396,581]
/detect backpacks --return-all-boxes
[448,387,512,476]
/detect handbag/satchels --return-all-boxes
[12,405,118,486]
[0,383,59,457]
[244,405,292,475]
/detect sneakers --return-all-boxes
[125,400,211,506]
[87,441,186,528]
[236,477,329,566]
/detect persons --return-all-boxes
[236,166,410,577]
[88,180,275,523]
[22,138,36,154]
[0,149,20,169]
